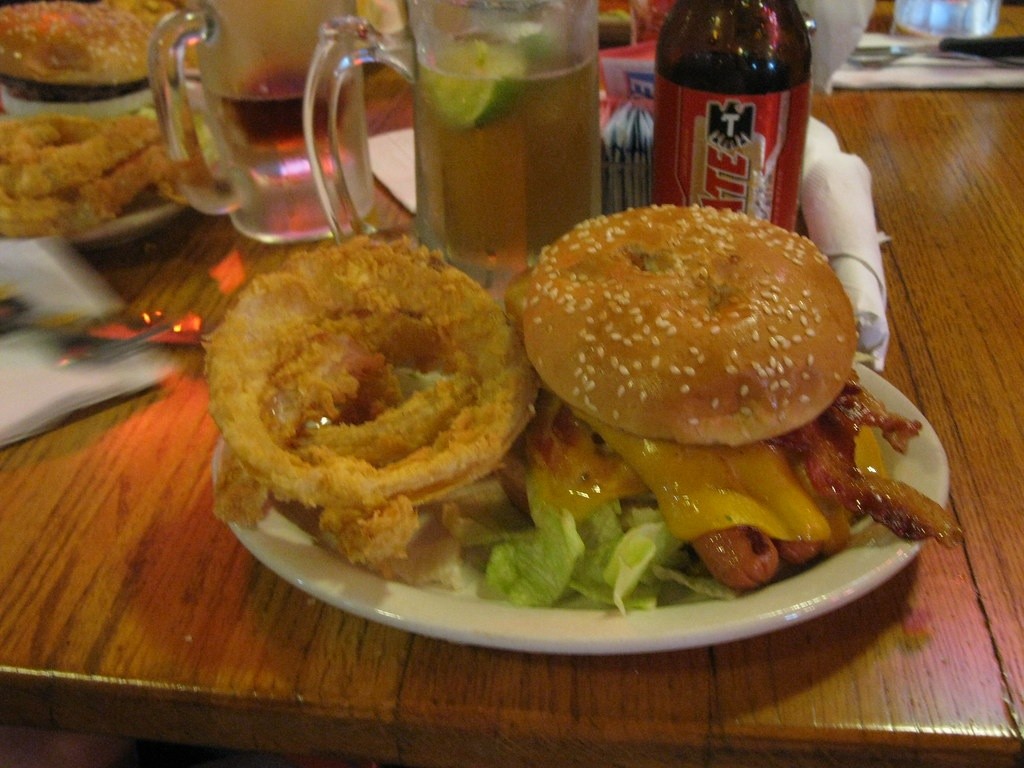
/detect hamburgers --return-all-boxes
[435,200,963,612]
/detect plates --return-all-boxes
[70,181,186,249]
[212,358,949,655]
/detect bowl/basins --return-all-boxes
[2,77,154,120]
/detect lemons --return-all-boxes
[419,41,520,129]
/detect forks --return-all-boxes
[847,35,1024,68]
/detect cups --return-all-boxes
[302,0,599,311]
[148,2,375,244]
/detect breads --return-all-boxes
[0,0,175,89]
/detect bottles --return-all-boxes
[651,0,812,232]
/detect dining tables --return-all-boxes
[0,0,1024,768]
